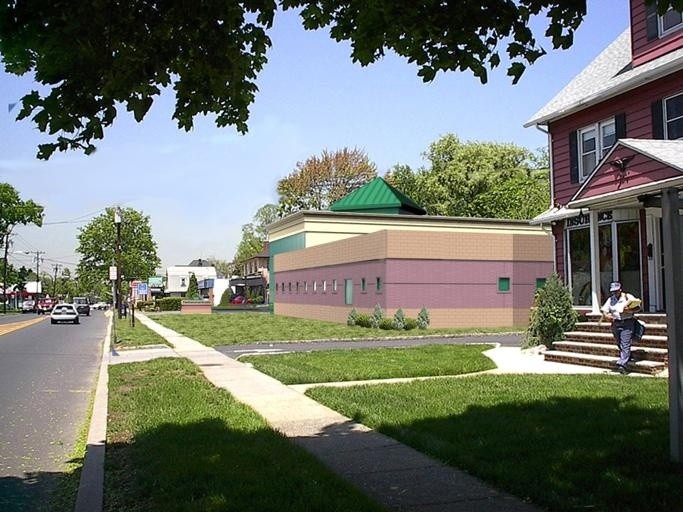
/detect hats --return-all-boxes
[609,283,621,292]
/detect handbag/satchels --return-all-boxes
[631,318,645,341]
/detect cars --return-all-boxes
[48,303,79,325]
[229,293,251,305]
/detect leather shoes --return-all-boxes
[618,366,629,374]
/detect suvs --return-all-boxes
[20,295,108,316]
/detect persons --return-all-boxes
[601,281,642,374]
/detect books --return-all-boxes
[625,298,641,309]
